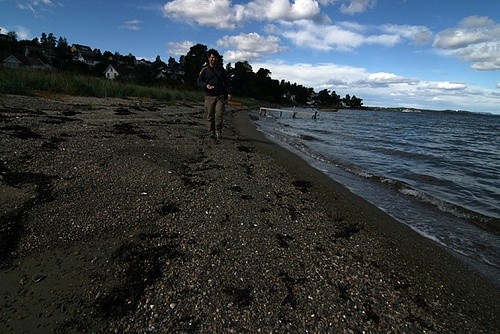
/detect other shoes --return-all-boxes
[215,131,222,140]
[209,133,215,138]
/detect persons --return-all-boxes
[198,51,231,139]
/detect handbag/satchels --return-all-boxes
[224,86,228,101]
